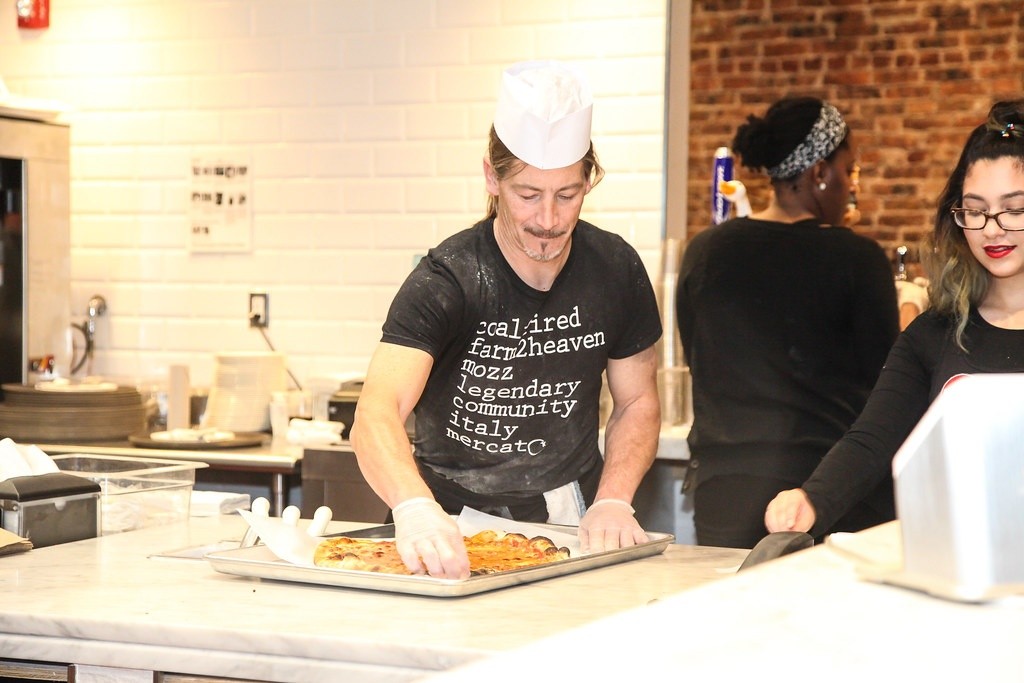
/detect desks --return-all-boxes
[27,439,301,520]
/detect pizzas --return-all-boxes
[313,529,572,578]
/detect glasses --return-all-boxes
[950,199,1024,231]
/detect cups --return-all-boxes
[289,387,313,421]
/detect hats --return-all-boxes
[493,61,593,170]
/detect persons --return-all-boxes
[764,94,1024,533]
[351,61,664,577]
[674,95,902,549]
